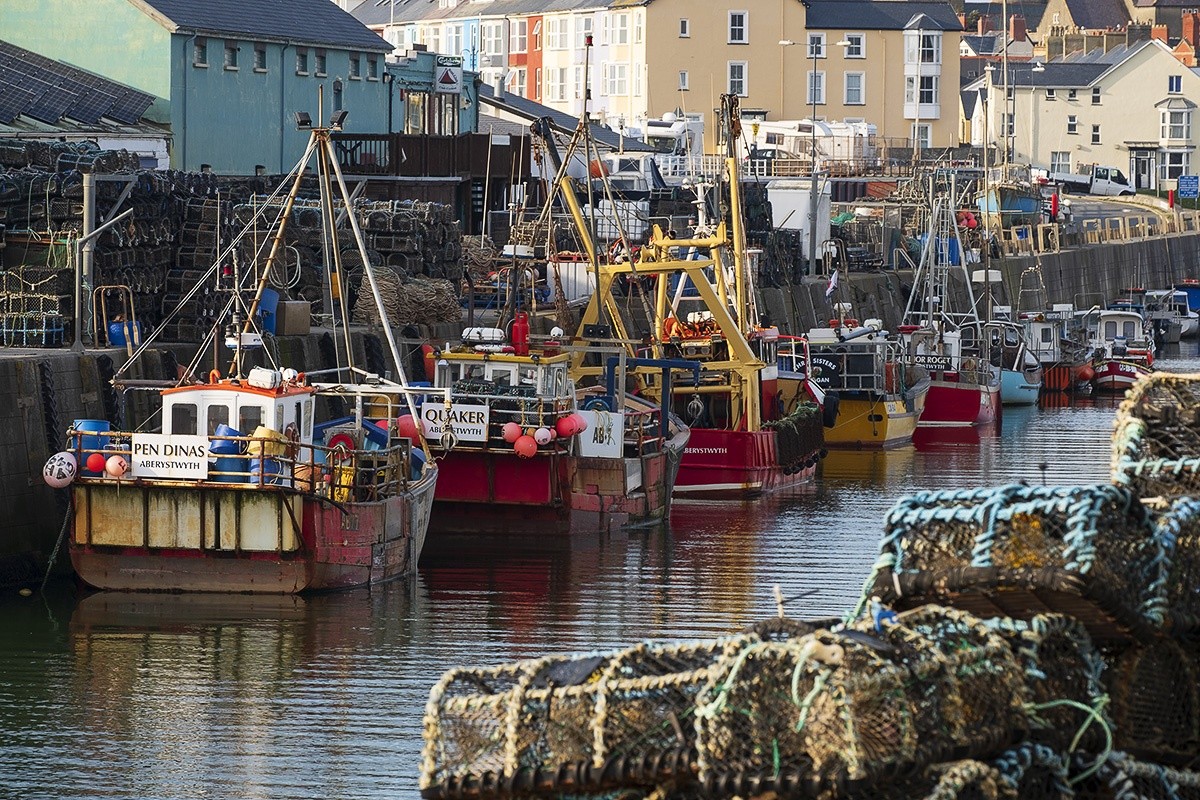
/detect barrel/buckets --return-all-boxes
[940,236,960,266]
[255,287,280,336]
[107,321,141,346]
[401,381,431,405]
[74,420,110,476]
[208,423,289,485]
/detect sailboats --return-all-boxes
[969,0,1044,237]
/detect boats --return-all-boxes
[40,81,461,598]
[397,27,703,534]
[570,83,1200,505]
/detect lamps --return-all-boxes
[1115,145,1120,149]
[383,72,391,84]
[305,166,312,175]
[1076,145,1080,149]
[256,165,266,176]
[201,164,212,173]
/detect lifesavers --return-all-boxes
[1018,314,1044,321]
[1182,279,1198,283]
[896,325,924,333]
[823,396,837,428]
[1114,299,1134,304]
[284,421,300,466]
[328,433,354,460]
[829,319,858,328]
[475,344,516,356]
[1147,350,1152,365]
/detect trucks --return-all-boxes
[1048,163,1137,197]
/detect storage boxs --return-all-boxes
[276,301,311,337]
[106,320,141,347]
[687,311,714,323]
[247,368,283,388]
[503,245,535,259]
[864,319,883,331]
[461,328,505,342]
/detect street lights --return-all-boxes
[777,40,852,276]
[983,65,1047,164]
